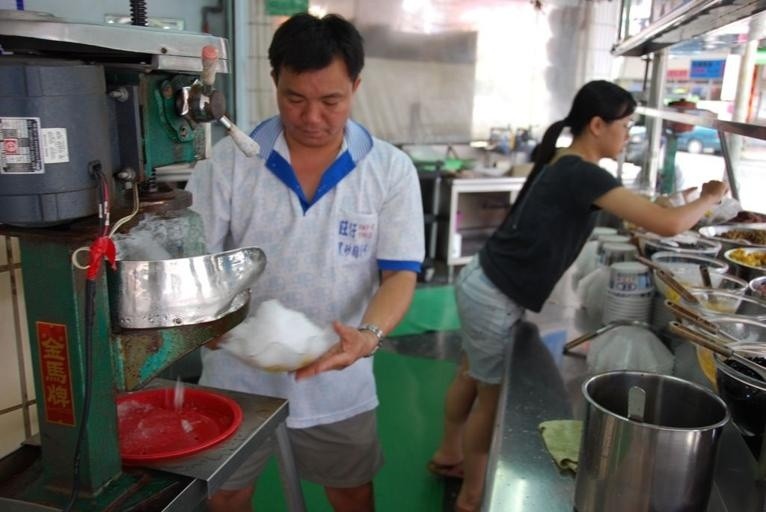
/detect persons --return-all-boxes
[433,78,727,511]
[176,7,428,512]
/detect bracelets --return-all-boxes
[355,322,387,359]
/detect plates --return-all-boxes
[209,298,341,373]
[587,326,676,378]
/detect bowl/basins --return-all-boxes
[635,222,766,448]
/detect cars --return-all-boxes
[675,125,730,157]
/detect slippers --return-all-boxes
[426,454,465,479]
